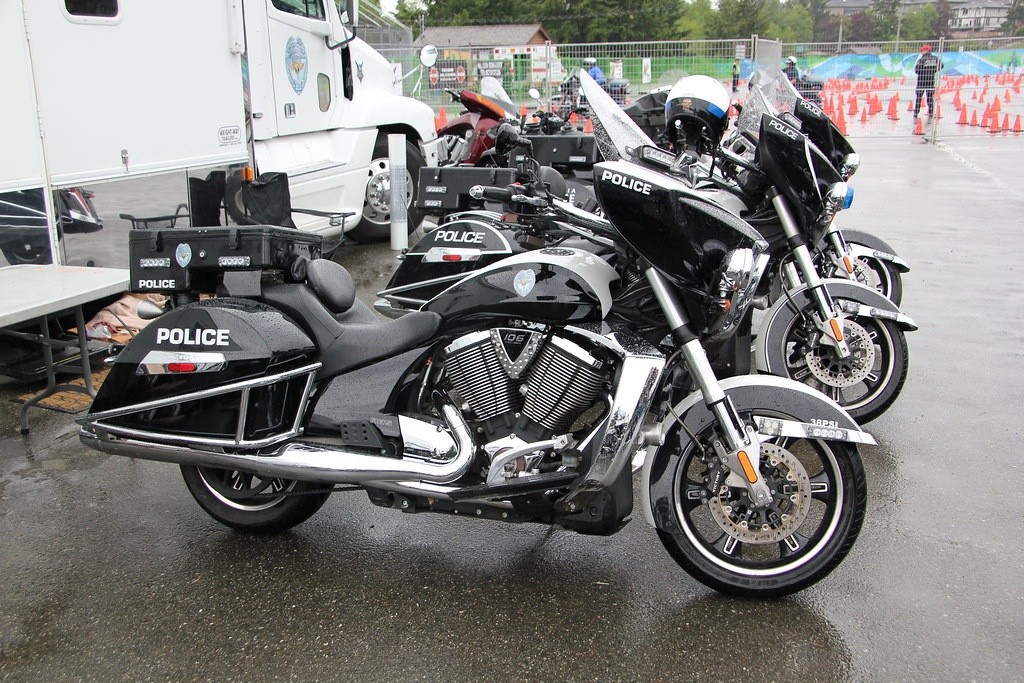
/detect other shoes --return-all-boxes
[929,115,933,117]
[913,114,917,117]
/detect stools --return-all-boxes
[119,171,228,232]
[238,171,355,265]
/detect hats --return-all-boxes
[919,45,931,50]
[735,57,740,59]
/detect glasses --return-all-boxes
[921,50,923,52]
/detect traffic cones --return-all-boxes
[439,105,447,129]
[434,115,441,134]
[520,102,527,115]
[584,117,593,133]
[820,74,1024,136]
[570,111,575,122]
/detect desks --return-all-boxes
[0,264,130,437]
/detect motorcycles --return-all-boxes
[1,189,102,267]
[82,60,920,597]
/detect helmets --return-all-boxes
[786,56,797,66]
[665,75,730,149]
[583,58,596,66]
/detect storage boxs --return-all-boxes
[416,166,517,209]
[127,224,324,291]
[802,75,825,88]
[609,77,630,93]
[509,134,597,171]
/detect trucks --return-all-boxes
[0,0,444,269]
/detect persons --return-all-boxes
[733,59,740,92]
[781,56,799,88]
[583,58,605,85]
[913,46,944,123]
